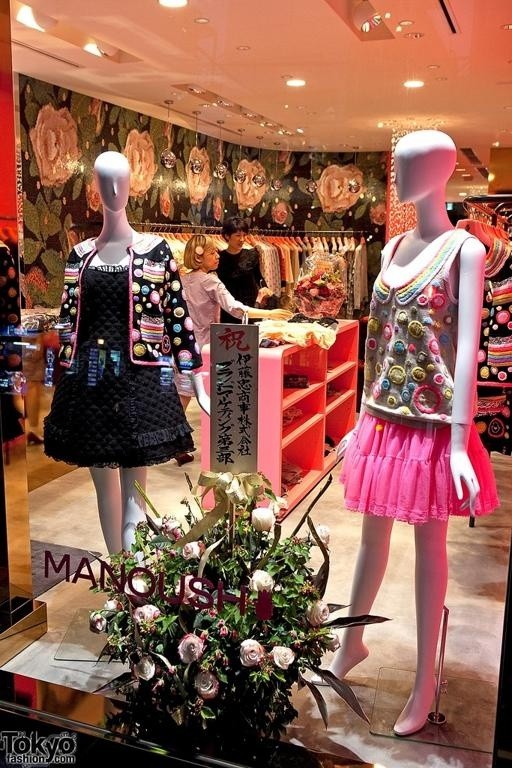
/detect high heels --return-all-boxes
[27,431,44,446]
[175,451,194,466]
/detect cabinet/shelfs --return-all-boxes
[198,320,362,528]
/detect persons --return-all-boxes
[44,150,203,611]
[208,215,269,325]
[312,127,503,738]
[173,234,294,467]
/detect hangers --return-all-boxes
[455,195,510,247]
[147,223,367,253]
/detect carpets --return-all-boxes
[31,539,103,602]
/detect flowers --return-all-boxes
[94,468,392,752]
